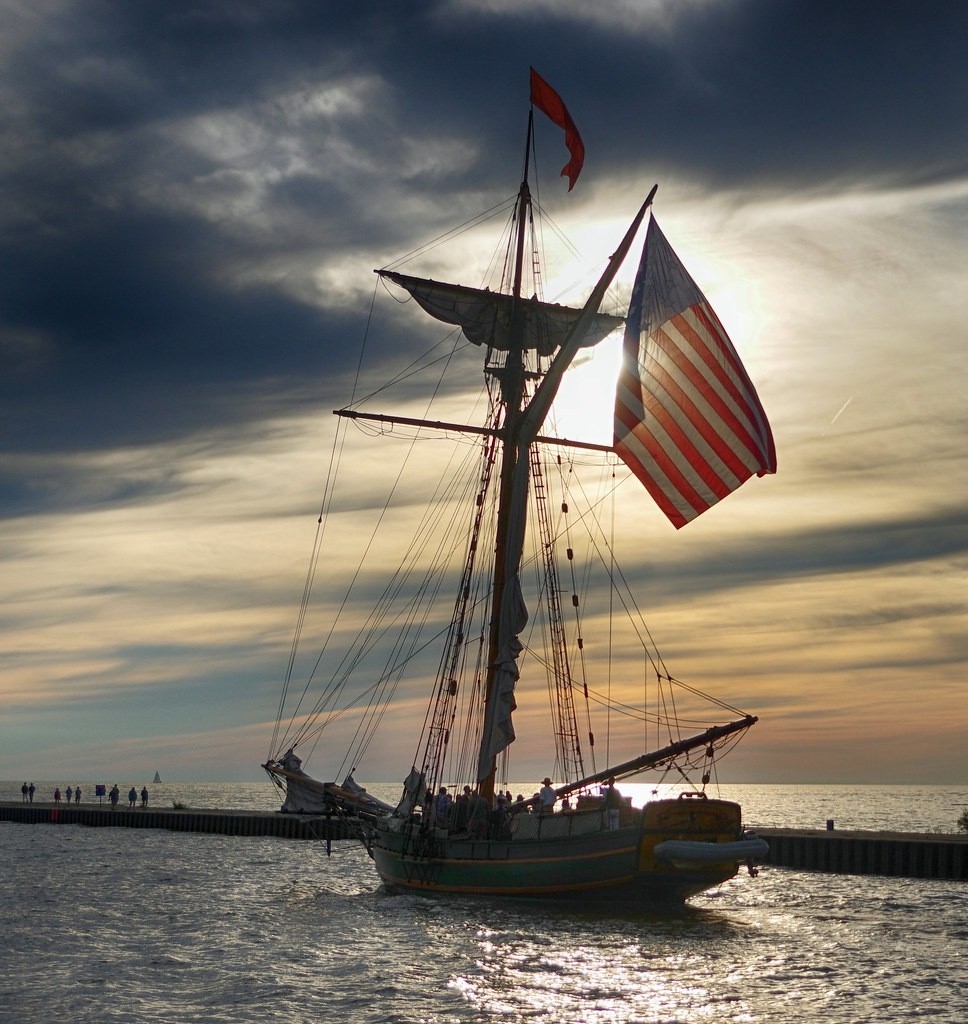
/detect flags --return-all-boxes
[611,211,778,531]
[531,69,584,192]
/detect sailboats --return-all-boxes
[153,771,162,783]
[262,64,770,905]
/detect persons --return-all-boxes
[491,798,507,826]
[497,791,505,797]
[434,787,451,827]
[75,787,81,804]
[506,791,512,805]
[54,788,61,803]
[109,787,118,808]
[539,778,556,814]
[514,795,529,813]
[22,782,28,803]
[141,786,148,806]
[29,783,35,803]
[114,784,119,794]
[66,785,73,805]
[446,786,490,817]
[129,787,137,807]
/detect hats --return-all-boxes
[541,778,553,784]
[517,795,525,799]
